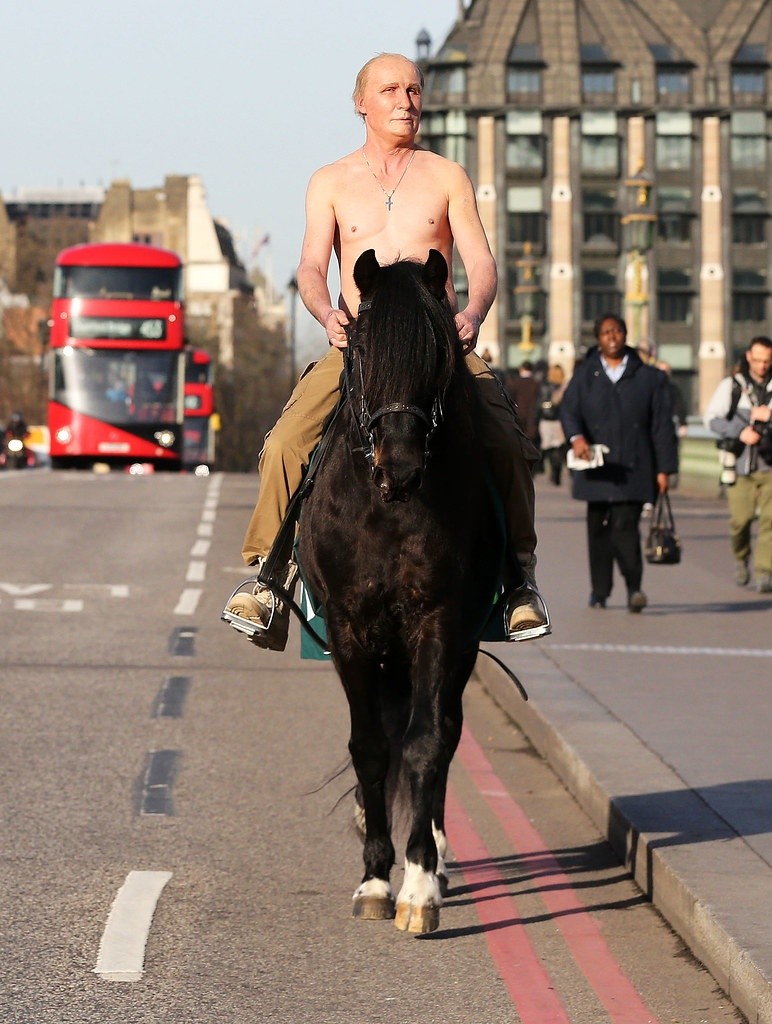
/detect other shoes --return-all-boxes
[628,591,647,613]
[590,600,606,608]
[756,582,772,592]
[736,561,749,585]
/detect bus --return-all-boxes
[43,240,191,472]
[183,346,222,471]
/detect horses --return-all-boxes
[289,249,500,933]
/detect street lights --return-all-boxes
[511,241,545,362]
[616,157,658,350]
[286,274,300,397]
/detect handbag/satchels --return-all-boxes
[645,491,681,564]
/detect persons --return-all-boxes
[505,362,567,485]
[705,337,772,592]
[558,314,682,613]
[221,54,555,639]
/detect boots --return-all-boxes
[508,554,547,631]
[226,559,298,651]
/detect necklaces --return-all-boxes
[361,144,416,213]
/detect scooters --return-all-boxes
[5,429,27,469]
